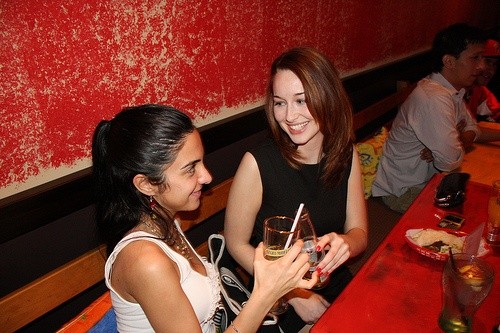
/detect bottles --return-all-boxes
[294,208,329,290]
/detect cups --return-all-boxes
[438,253,494,333]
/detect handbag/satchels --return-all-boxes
[207,233,284,333]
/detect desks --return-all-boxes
[308,118,500,333]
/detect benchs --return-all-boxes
[0,85,417,333]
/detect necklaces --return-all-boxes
[141,213,193,261]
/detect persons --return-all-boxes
[90,103,318,333]
[373,22,487,213]
[463,39,500,143]
[224,46,368,333]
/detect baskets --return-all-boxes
[400,226,494,262]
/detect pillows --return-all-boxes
[349,129,387,201]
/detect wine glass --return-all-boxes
[262,217,302,315]
[481,179,500,246]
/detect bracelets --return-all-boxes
[231,322,239,333]
